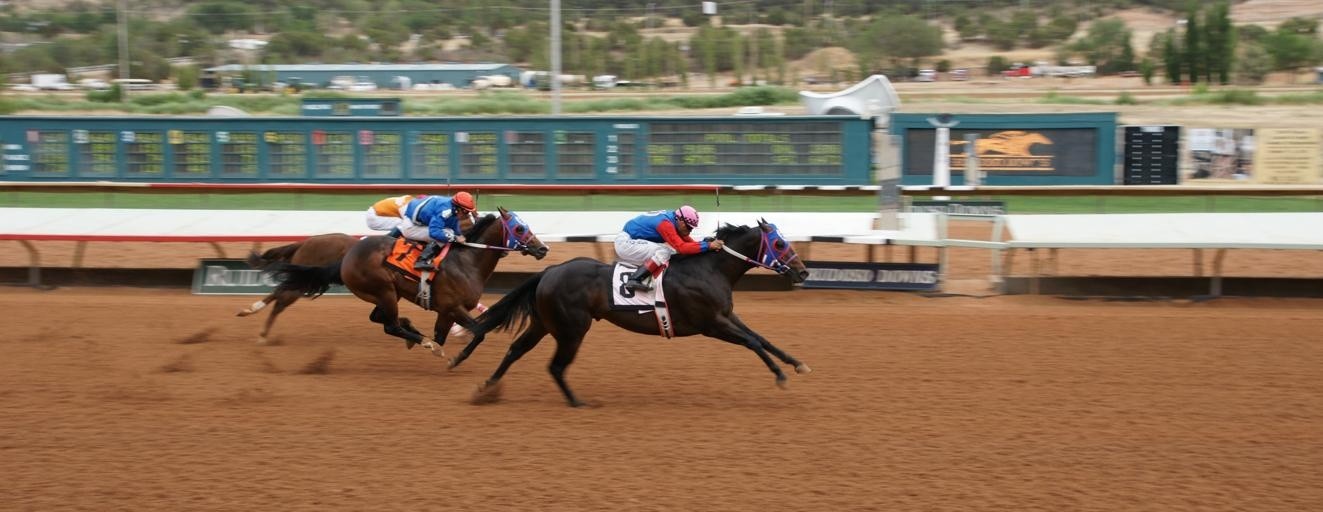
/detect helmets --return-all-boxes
[676,206,698,226]
[452,191,476,212]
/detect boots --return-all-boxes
[626,265,653,290]
[414,241,440,269]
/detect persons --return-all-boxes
[400,192,477,271]
[613,206,724,291]
[365,195,429,237]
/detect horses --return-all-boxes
[262,206,549,371]
[236,234,488,344]
[464,218,812,407]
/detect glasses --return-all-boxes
[679,208,692,230]
[455,192,468,214]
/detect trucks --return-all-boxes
[1002,63,1096,79]
[29,73,70,91]
[470,73,511,91]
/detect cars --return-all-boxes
[918,70,936,82]
[80,78,110,89]
[952,70,971,79]
